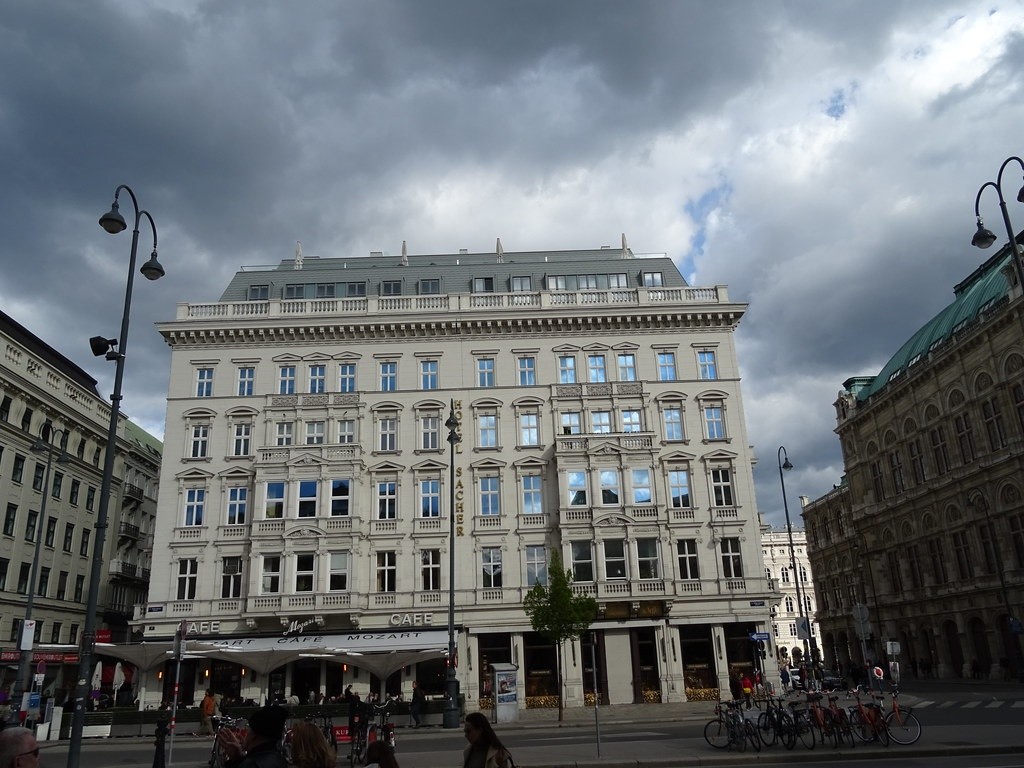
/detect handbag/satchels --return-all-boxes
[743,687,751,694]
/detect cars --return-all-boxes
[789,669,804,689]
[821,669,848,690]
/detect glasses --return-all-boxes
[464,728,474,734]
[19,748,39,758]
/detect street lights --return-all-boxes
[777,445,815,677]
[970,155,1024,297]
[64,184,167,768]
[440,396,463,729]
[6,421,70,729]
[964,484,1024,682]
[789,556,818,680]
[852,532,892,681]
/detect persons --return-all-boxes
[45,689,51,698]
[0,726,41,768]
[193,681,425,768]
[729,653,1024,712]
[462,712,516,768]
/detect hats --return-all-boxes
[248,705,289,740]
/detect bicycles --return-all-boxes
[702,683,922,753]
[209,697,396,768]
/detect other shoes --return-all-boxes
[206,734,214,738]
[413,726,420,729]
[415,722,423,728]
[193,732,200,737]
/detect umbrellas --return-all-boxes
[113,663,126,707]
[293,240,304,270]
[93,661,102,710]
[497,238,504,263]
[622,233,628,258]
[131,668,139,703]
[402,241,409,266]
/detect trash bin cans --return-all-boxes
[443,704,460,728]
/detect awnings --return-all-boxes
[194,631,458,652]
